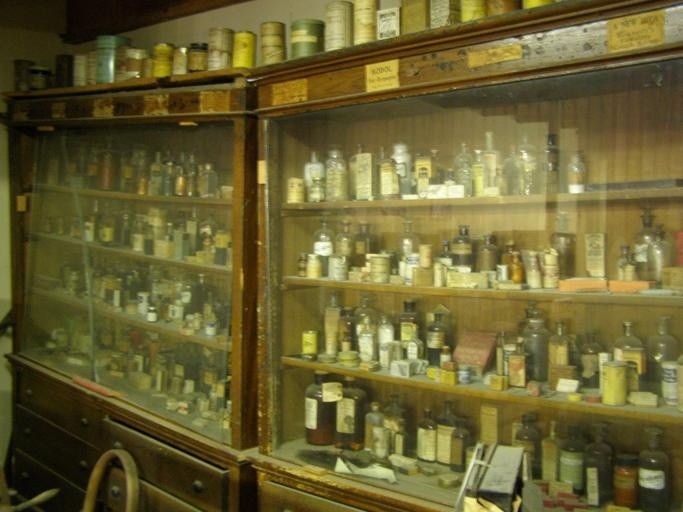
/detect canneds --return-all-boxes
[660,361,678,406]
[304,253,324,277]
[302,329,318,355]
[308,176,324,199]
[602,361,627,407]
[327,253,351,280]
[370,254,391,284]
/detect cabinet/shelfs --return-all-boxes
[246,0,683,512]
[2,67,257,512]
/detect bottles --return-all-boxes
[287,131,683,510]
[105,342,233,446]
[27,131,230,340]
